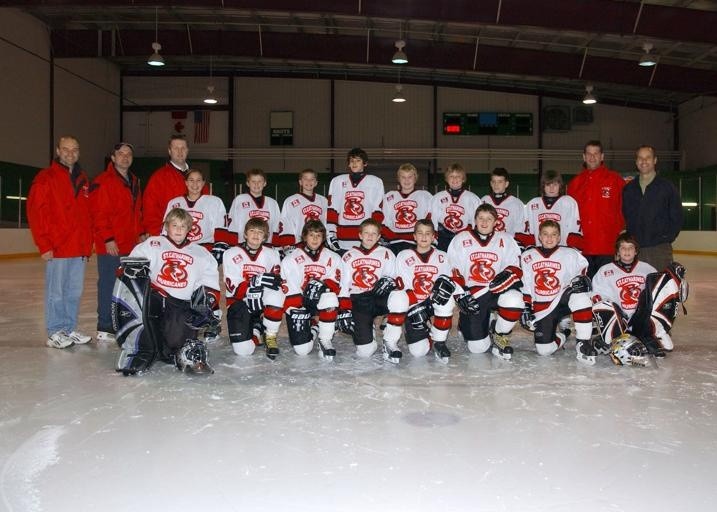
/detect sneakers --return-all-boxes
[490,330,514,354]
[318,338,336,357]
[47,332,116,349]
[576,341,597,356]
[382,338,403,358]
[204,323,221,339]
[433,341,451,358]
[264,332,279,358]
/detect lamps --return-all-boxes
[145,4,167,67]
[637,39,658,67]
[391,3,410,104]
[581,79,598,104]
[202,55,218,105]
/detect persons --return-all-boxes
[227,168,281,247]
[86,143,141,343]
[397,219,455,362]
[428,163,481,253]
[380,163,433,257]
[515,221,598,367]
[279,169,328,255]
[564,137,628,279]
[162,169,228,322]
[619,143,682,269]
[27,131,96,348]
[447,204,525,360]
[326,149,384,257]
[582,234,689,368]
[342,218,409,364]
[133,132,209,237]
[111,204,218,381]
[224,218,285,360]
[281,220,352,362]
[520,170,587,252]
[481,168,526,253]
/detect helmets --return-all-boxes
[610,335,653,367]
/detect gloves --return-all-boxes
[290,310,313,334]
[429,273,480,316]
[570,276,591,292]
[300,279,326,310]
[408,303,432,332]
[373,278,396,298]
[246,290,264,313]
[489,268,523,294]
[252,274,283,290]
[336,309,355,335]
